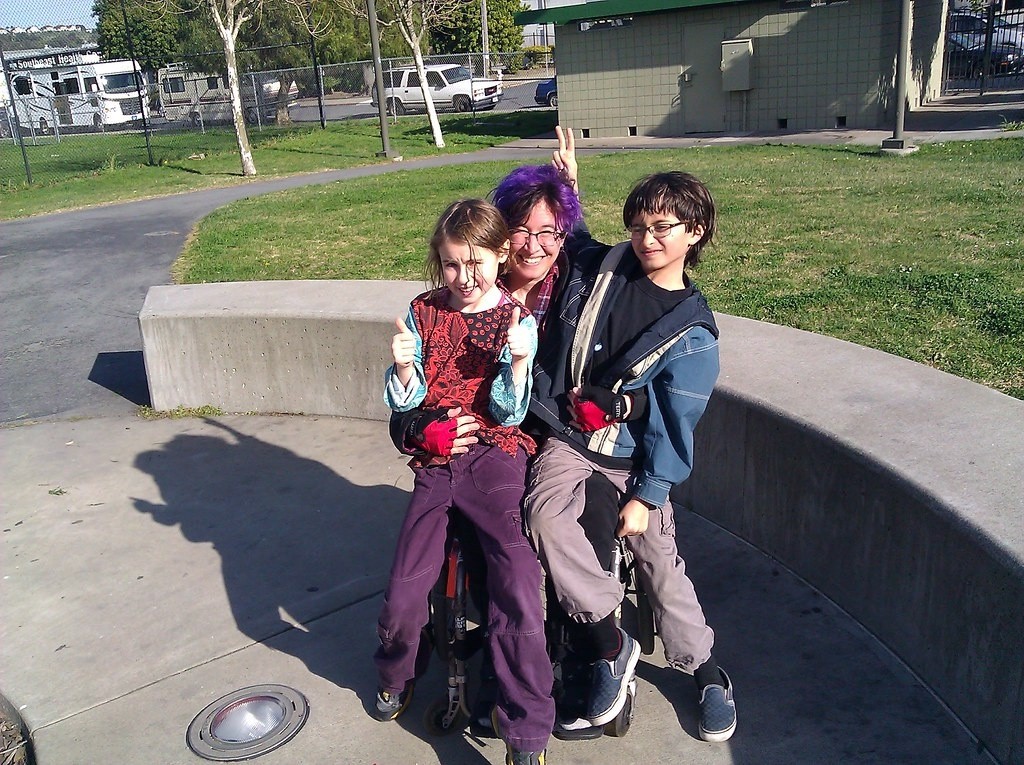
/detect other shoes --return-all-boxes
[478,655,501,728]
[697,666,738,742]
[587,626,641,726]
[548,642,593,731]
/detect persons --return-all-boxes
[388,164,615,740]
[375,199,556,765]
[523,124,736,744]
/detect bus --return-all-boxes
[157,61,299,126]
[2,58,151,136]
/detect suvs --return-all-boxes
[371,64,504,116]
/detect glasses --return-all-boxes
[623,220,690,240]
[508,228,566,245]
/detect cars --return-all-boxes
[536,78,558,107]
[947,12,1024,50]
[942,30,1024,79]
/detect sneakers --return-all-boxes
[374,676,417,722]
[490,706,545,765]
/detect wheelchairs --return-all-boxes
[424,494,656,738]
